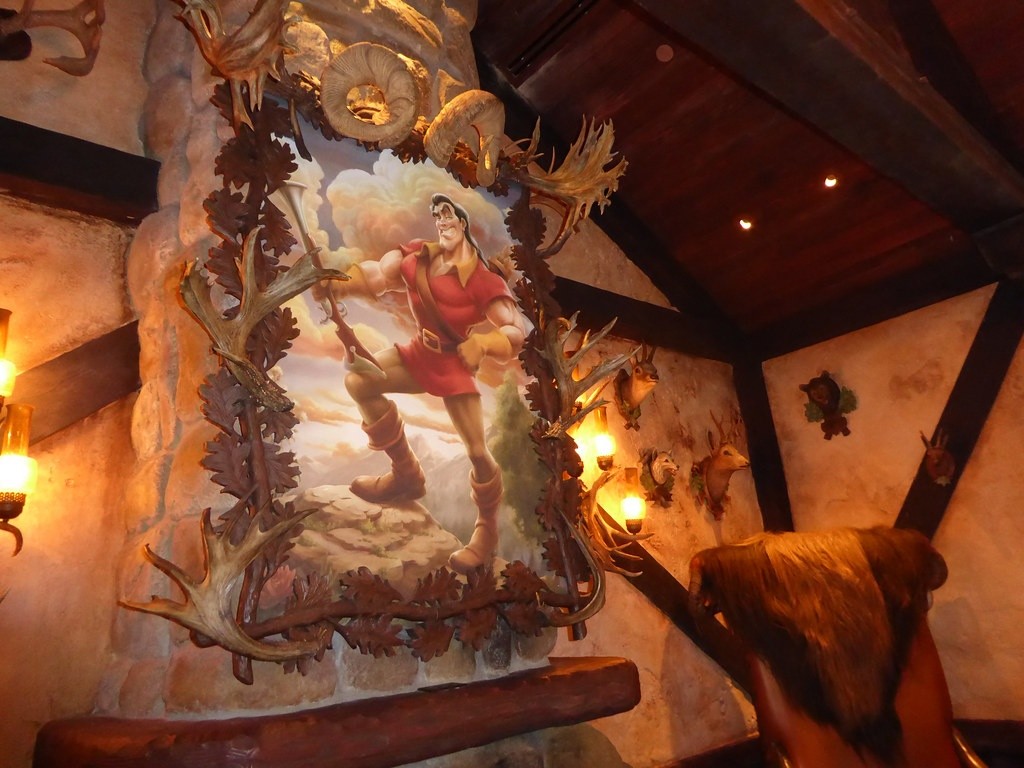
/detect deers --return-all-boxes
[613,338,659,432]
[691,409,751,521]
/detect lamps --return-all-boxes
[0,453,35,557]
[596,433,615,471]
[624,495,643,535]
[0,358,16,413]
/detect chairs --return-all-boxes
[688,524,989,768]
